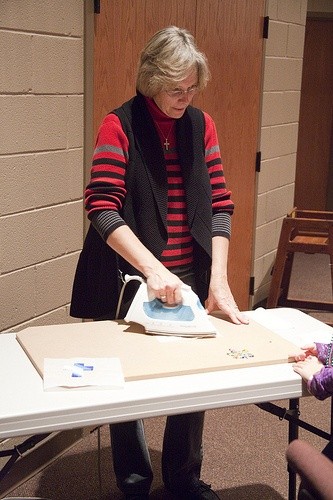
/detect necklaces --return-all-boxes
[147,105,176,151]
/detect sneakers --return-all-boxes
[125,494,150,500]
[172,478,223,500]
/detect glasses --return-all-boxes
[166,87,200,98]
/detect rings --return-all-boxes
[159,294,165,299]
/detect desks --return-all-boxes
[0,308,333,500]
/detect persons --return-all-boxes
[70,27,250,500]
[293,328,333,420]
